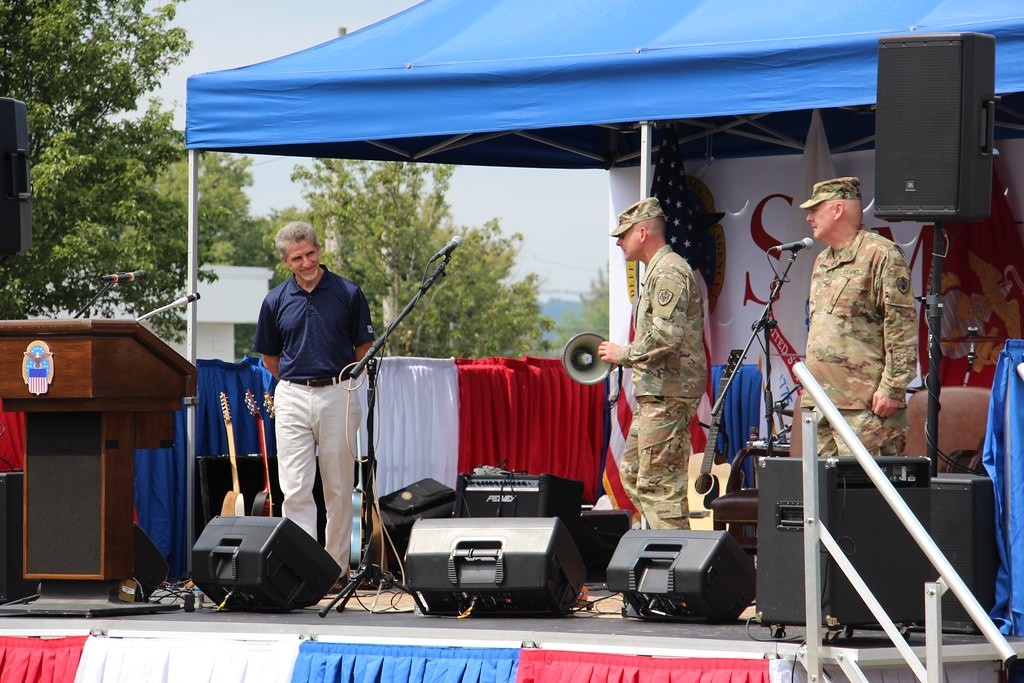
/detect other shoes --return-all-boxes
[327,573,349,594]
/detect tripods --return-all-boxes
[316,255,451,617]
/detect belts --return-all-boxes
[285,374,350,387]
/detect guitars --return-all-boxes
[349,423,389,573]
[684,349,747,533]
[219,387,284,518]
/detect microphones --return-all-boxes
[101,270,147,283]
[768,238,815,252]
[430,236,464,261]
[137,293,201,323]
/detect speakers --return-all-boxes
[874,32,995,223]
[0,97,33,258]
[0,472,40,603]
[130,521,169,603]
[403,473,631,620]
[606,528,757,624]
[758,456,1000,633]
[193,515,342,612]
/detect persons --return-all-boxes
[597,198,708,532]
[252,222,374,596]
[798,176,919,456]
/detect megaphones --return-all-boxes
[561,332,613,385]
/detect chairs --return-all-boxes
[898,387,990,474]
[710,394,803,555]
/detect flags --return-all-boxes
[755,108,840,469]
[650,121,704,274]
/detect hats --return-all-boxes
[609,196,664,237]
[799,176,862,210]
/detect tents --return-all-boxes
[184,0,1023,683]
